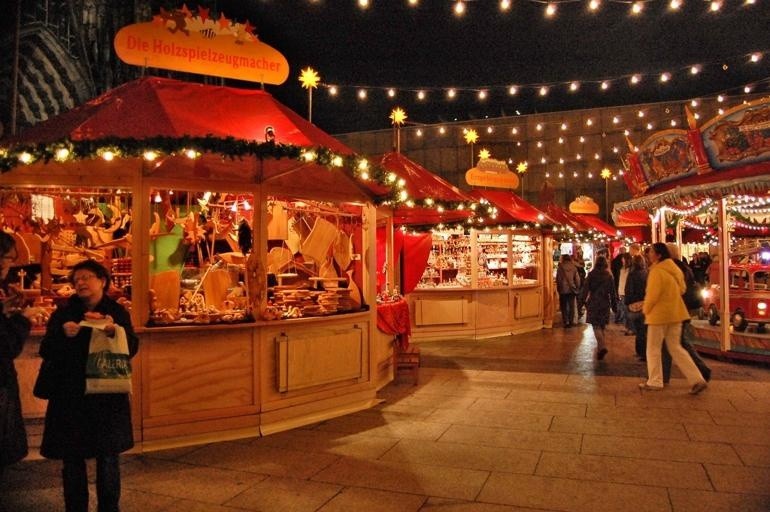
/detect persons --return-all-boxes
[555,241,714,397]
[0,227,38,470]
[31,258,142,512]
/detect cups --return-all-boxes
[71,274,102,284]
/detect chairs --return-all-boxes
[638,382,663,392]
[688,379,708,397]
[596,349,608,360]
[700,366,711,382]
[663,377,669,386]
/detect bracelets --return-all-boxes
[270,285,354,318]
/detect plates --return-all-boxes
[418,238,471,283]
[480,241,528,270]
